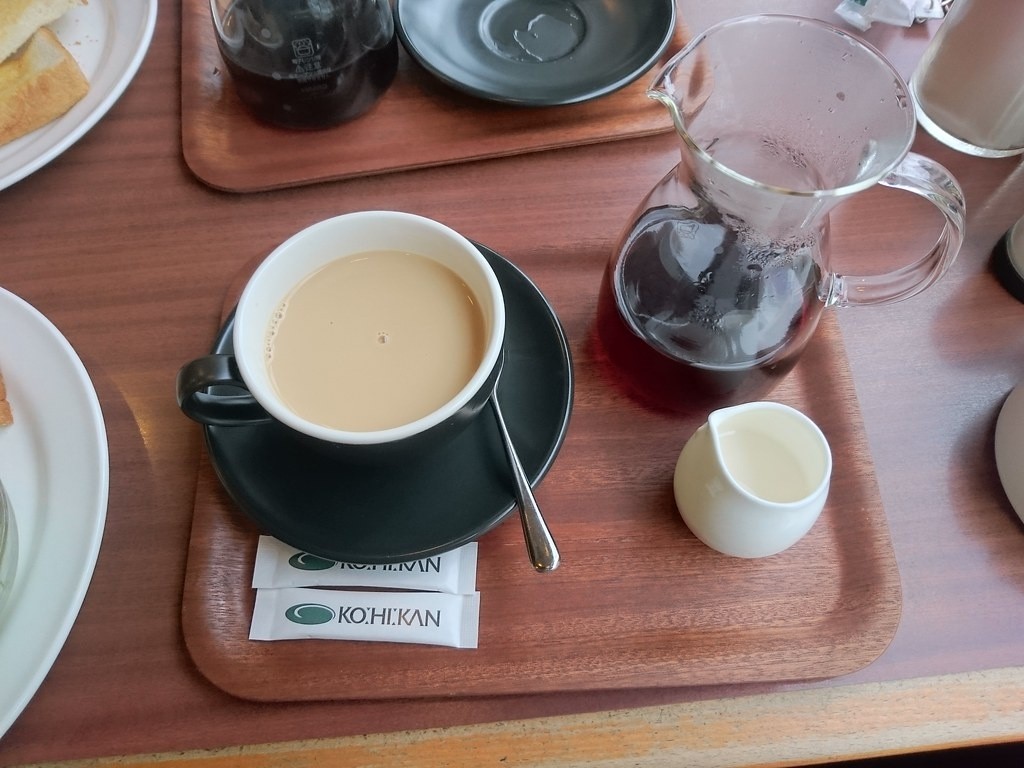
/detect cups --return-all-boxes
[994,382,1023,524]
[673,401,833,559]
[176,210,504,455]
[208,0,399,129]
[910,0,1024,162]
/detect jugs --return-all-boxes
[595,14,974,416]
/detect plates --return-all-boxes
[194,242,573,561]
[0,0,160,194]
[395,0,676,108]
[1,290,109,751]
